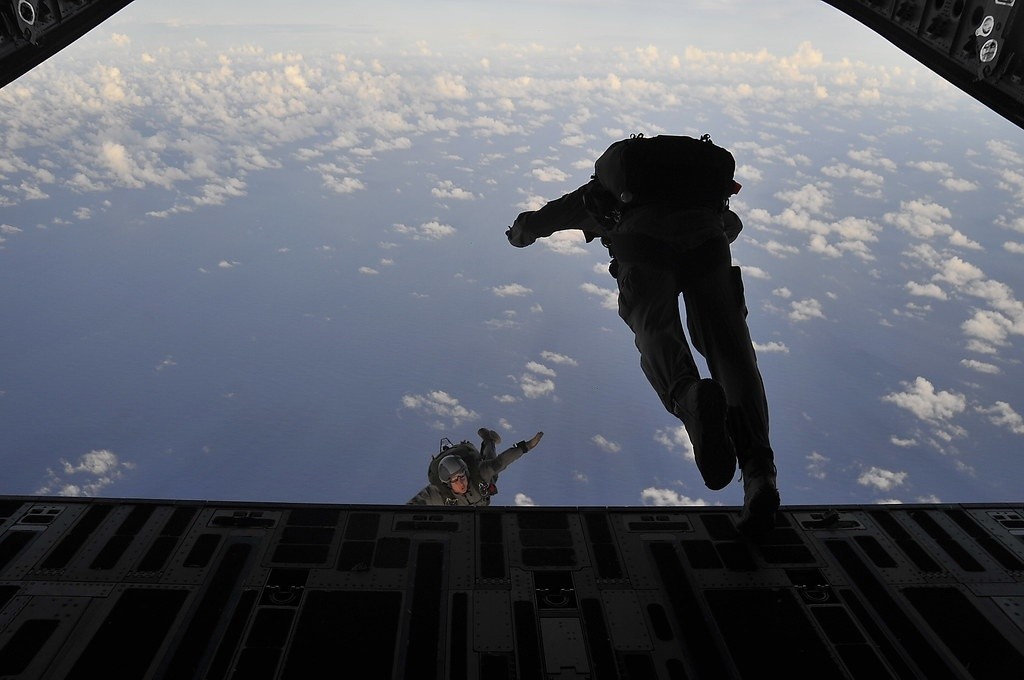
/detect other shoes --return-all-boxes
[478,429,501,444]
[671,377,736,490]
[734,475,780,538]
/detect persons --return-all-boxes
[505,180,781,506]
[406,427,543,506]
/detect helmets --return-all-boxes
[438,455,470,488]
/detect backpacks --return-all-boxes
[428,441,480,486]
[594,133,735,212]
